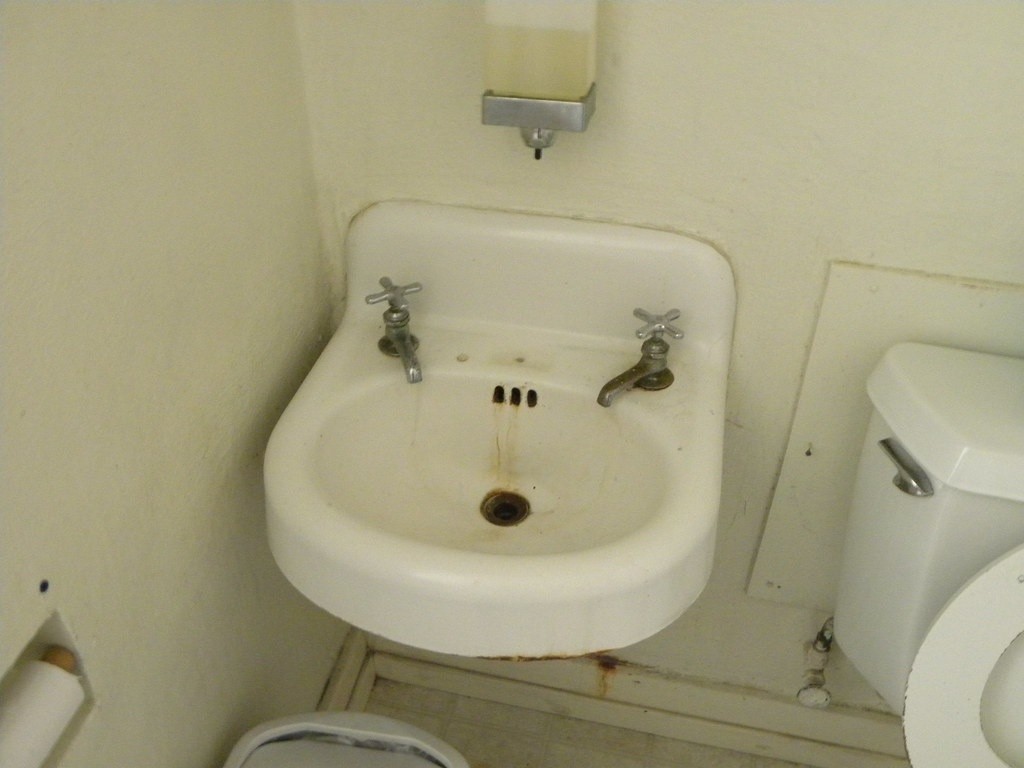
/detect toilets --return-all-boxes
[829,338,1024,768]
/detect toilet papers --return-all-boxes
[1,657,86,767]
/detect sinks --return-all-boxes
[259,198,738,662]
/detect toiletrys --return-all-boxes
[481,1,599,102]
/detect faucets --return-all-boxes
[594,307,687,410]
[364,275,425,385]
